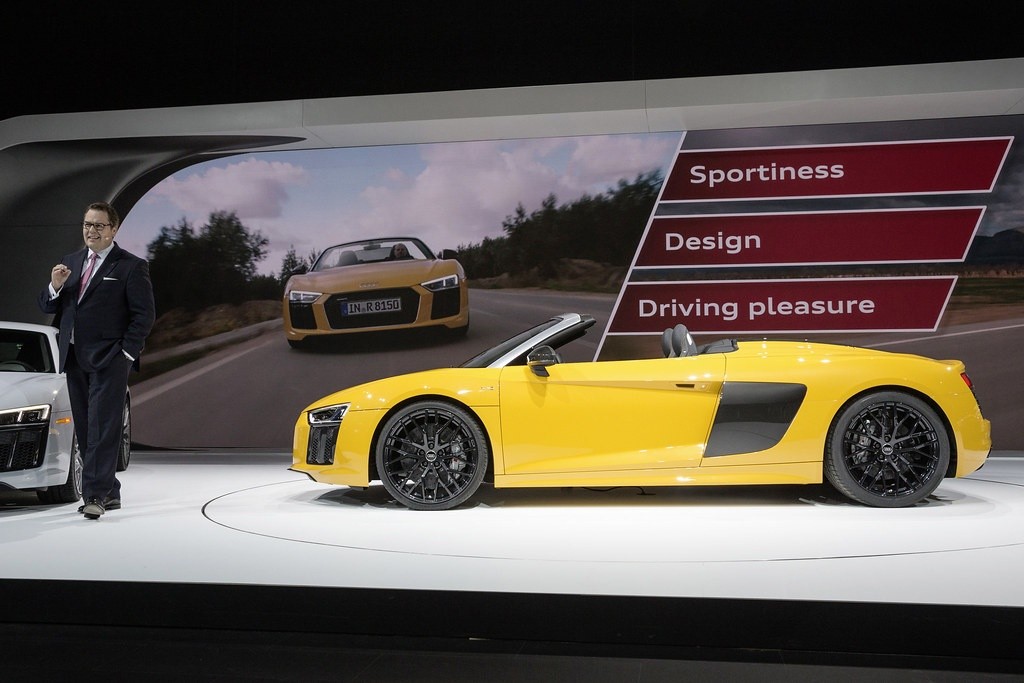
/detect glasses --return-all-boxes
[81,222,111,230]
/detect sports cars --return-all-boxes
[282,236,472,351]
[285,310,994,509]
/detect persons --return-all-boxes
[38,202,156,519]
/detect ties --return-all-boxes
[79,253,100,295]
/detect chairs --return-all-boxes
[661,328,678,358]
[337,250,364,267]
[672,324,698,358]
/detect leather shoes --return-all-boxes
[83,497,105,519]
[77,496,121,512]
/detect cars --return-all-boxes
[0,320,132,504]
